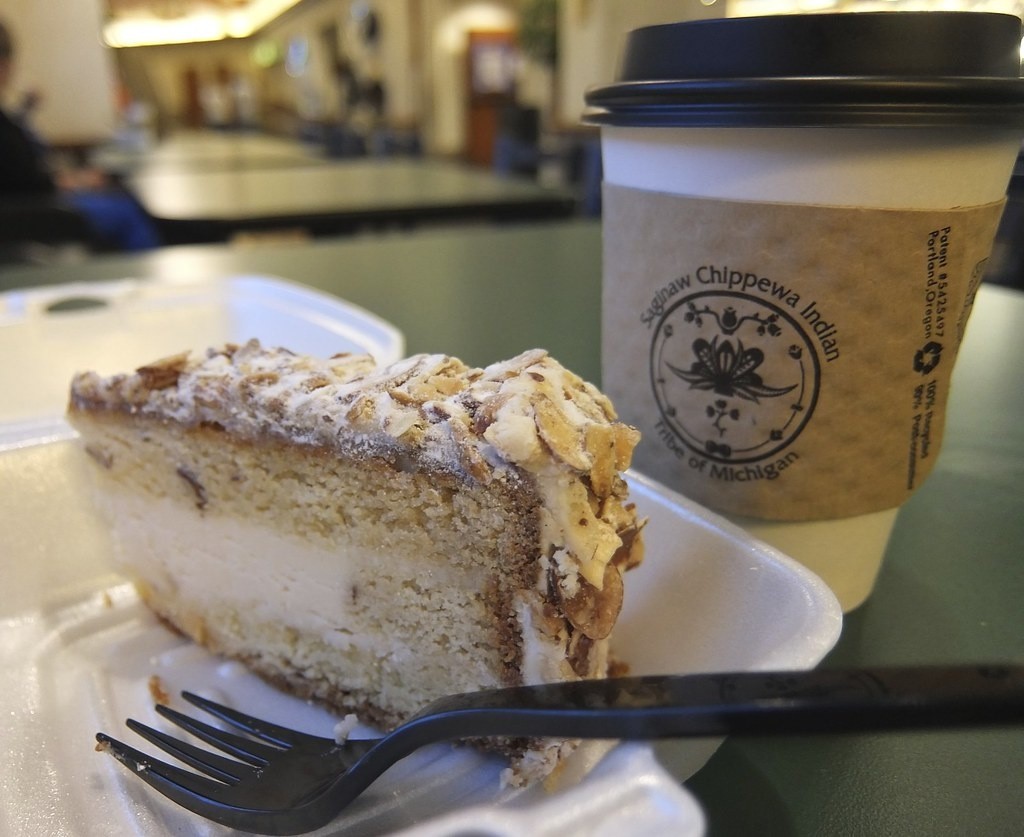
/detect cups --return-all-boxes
[579,10,1021,614]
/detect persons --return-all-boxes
[0,16,139,263]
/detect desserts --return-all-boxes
[64,339,649,791]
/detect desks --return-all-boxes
[0,226,1024,835]
[115,154,584,224]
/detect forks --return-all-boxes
[92,658,1024,834]
